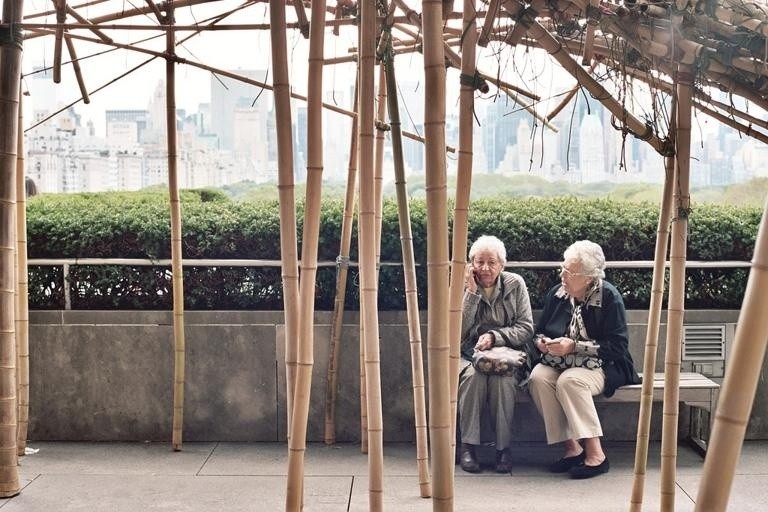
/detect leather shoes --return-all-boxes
[459,442,479,472]
[496,449,513,473]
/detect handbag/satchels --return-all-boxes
[472,345,528,377]
[540,347,604,372]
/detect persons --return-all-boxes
[524,239,639,479]
[456,234,535,475]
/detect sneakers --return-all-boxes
[548,448,589,473]
[568,456,609,479]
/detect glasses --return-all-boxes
[560,265,586,277]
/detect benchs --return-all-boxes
[515,372,720,465]
